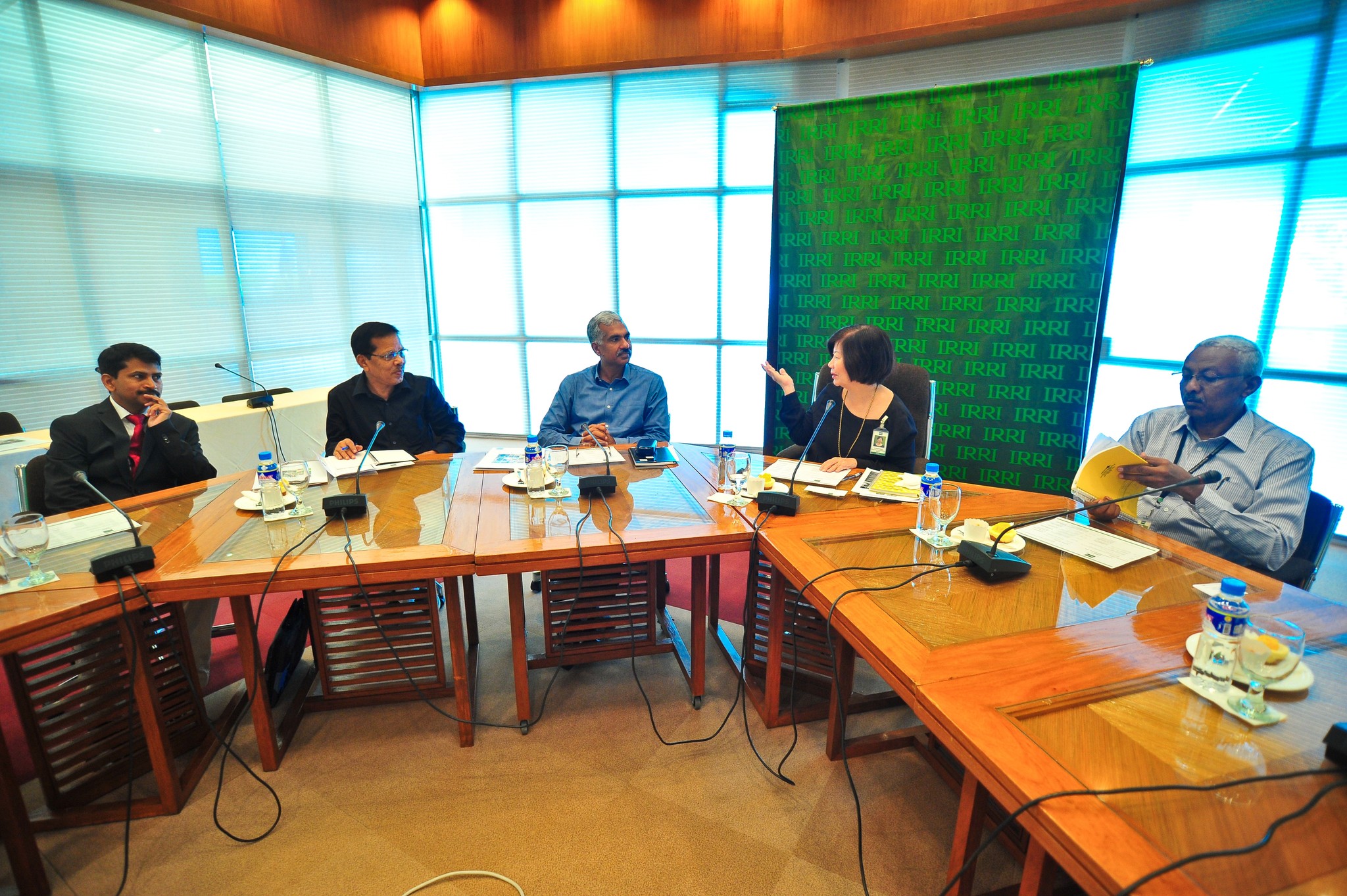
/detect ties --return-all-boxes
[126,415,146,478]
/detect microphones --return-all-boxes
[577,421,617,495]
[67,463,157,583]
[214,362,274,409]
[322,412,390,517]
[957,470,1222,584]
[757,389,840,516]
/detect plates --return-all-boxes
[732,480,789,498]
[1185,628,1316,690]
[950,523,1027,553]
[502,470,556,489]
[234,490,298,510]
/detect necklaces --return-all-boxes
[839,384,880,459]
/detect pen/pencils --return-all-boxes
[840,472,863,481]
[341,443,358,451]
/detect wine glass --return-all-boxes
[548,497,571,537]
[544,444,569,496]
[930,481,962,547]
[1228,614,1307,725]
[2,512,57,587]
[726,451,751,507]
[280,459,314,515]
[292,516,321,556]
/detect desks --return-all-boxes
[4,387,336,520]
[0,433,1347,896]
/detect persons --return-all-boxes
[324,321,441,613]
[37,344,217,768]
[873,436,885,448]
[760,325,918,474]
[538,310,670,671]
[1081,334,1315,573]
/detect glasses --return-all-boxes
[367,349,408,361]
[1171,370,1254,389]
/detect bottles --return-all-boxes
[717,430,736,491]
[915,462,943,540]
[264,519,290,552]
[527,497,546,547]
[1189,577,1251,693]
[525,434,545,497]
[257,450,286,522]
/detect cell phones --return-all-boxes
[636,438,658,457]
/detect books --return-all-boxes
[328,448,419,471]
[627,447,677,466]
[1070,432,1152,523]
[868,470,923,498]
[628,453,676,470]
[1059,550,1125,608]
